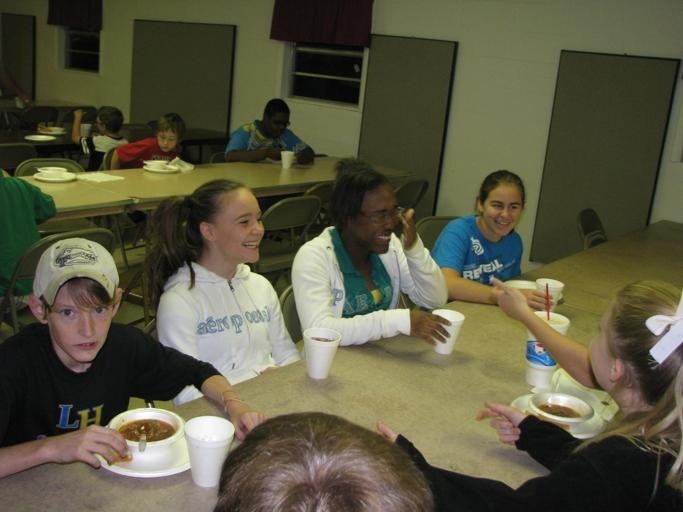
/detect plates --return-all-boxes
[24,136,55,143]
[142,165,179,174]
[91,427,191,479]
[38,127,68,135]
[503,280,563,304]
[33,173,77,183]
[509,394,604,440]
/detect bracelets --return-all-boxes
[221,390,245,412]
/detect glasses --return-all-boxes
[358,206,407,225]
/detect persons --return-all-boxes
[111,113,185,221]
[0,64,33,113]
[0,169,57,310]
[0,238,271,480]
[371,282,683,511]
[224,99,315,215]
[153,178,300,404]
[72,107,129,173]
[488,274,605,392]
[423,170,553,318]
[214,411,435,511]
[289,164,452,350]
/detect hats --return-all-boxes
[33,236,121,307]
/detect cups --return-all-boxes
[79,124,91,138]
[303,326,341,381]
[280,150,294,168]
[433,309,465,355]
[524,309,569,388]
[535,278,564,311]
[183,414,235,489]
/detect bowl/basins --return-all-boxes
[144,160,166,169]
[39,167,63,177]
[107,407,183,470]
[530,391,593,431]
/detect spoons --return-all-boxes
[133,421,153,452]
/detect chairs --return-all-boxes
[0,105,608,408]
